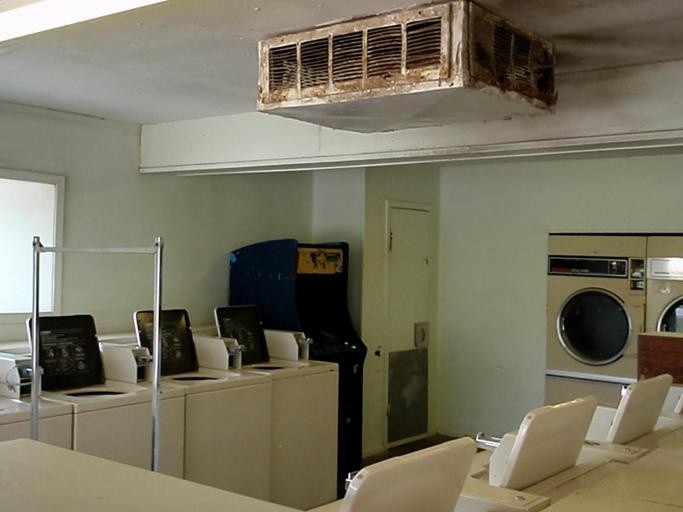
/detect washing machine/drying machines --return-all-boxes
[312,374,680,512]
[546,234,682,413]
[1,305,339,512]
